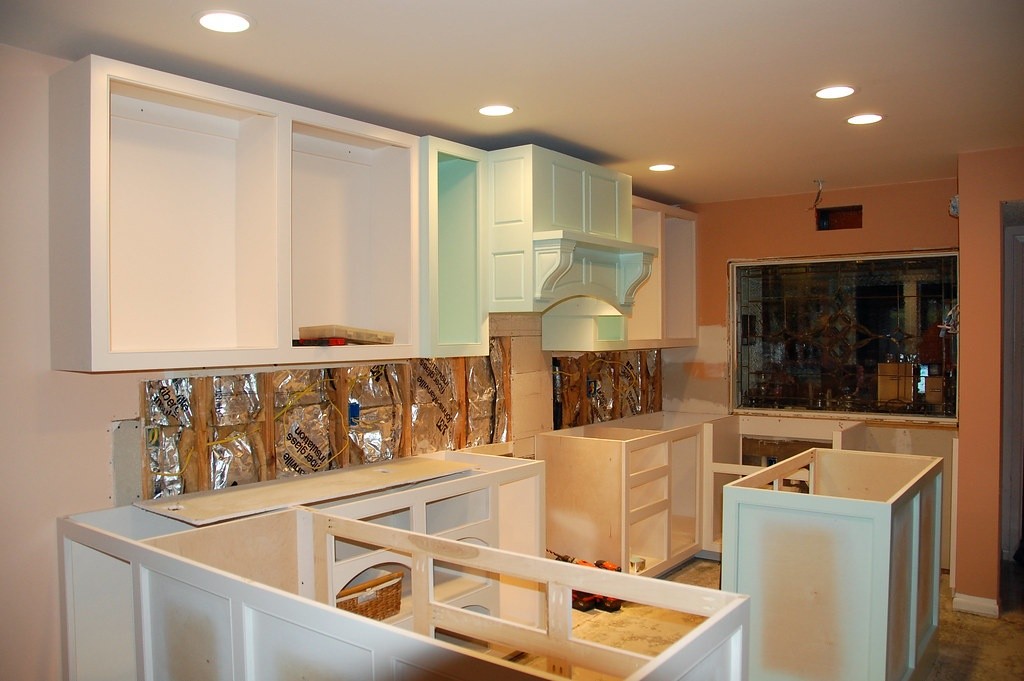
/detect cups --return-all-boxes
[630,558,645,572]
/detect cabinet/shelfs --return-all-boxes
[50,53,699,375]
[55,409,948,681]
[925,376,943,403]
[878,363,914,405]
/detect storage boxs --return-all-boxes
[299,324,395,344]
